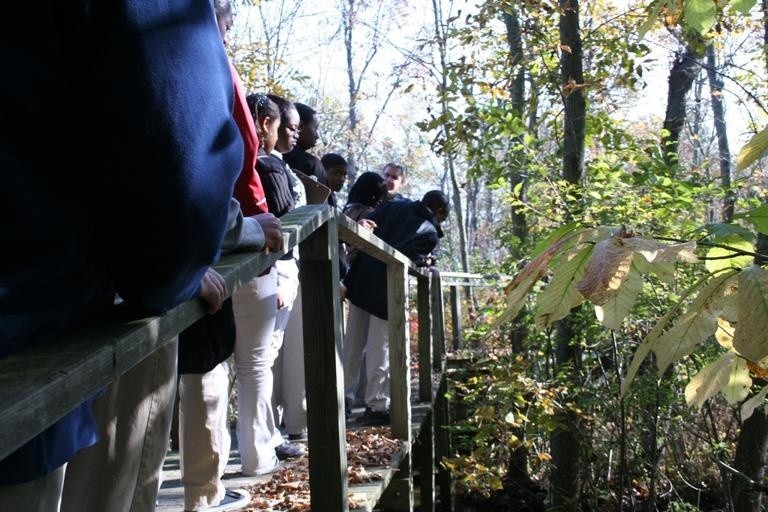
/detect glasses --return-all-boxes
[282,125,302,136]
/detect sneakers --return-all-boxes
[344,401,354,420]
[275,441,308,461]
[288,431,308,440]
[183,487,253,512]
[362,406,393,426]
